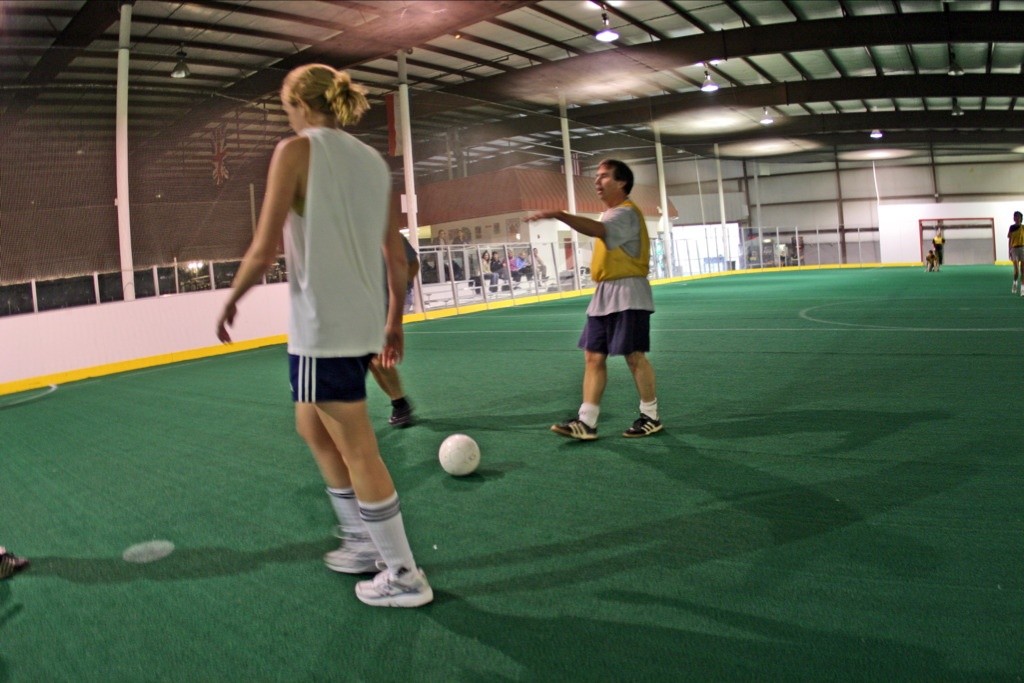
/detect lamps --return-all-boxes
[759,107,773,123]
[948,51,965,76]
[595,5,618,41]
[701,69,720,92]
[171,51,191,78]
[951,98,965,115]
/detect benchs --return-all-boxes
[418,271,550,308]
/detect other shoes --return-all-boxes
[0,551,28,580]
[1012,281,1018,294]
[1021,286,1024,296]
[387,400,411,426]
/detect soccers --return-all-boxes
[438,433,481,477]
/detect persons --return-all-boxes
[368,226,419,427]
[0,545,30,578]
[925,225,945,272]
[421,229,551,295]
[215,65,434,607]
[1008,211,1024,297]
[523,158,666,440]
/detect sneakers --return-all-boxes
[354,564,434,608]
[324,530,383,574]
[550,420,598,439]
[623,412,663,438]
